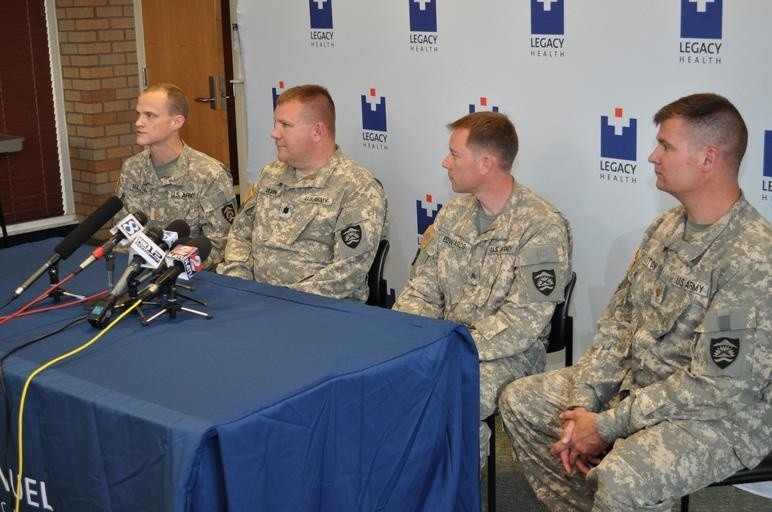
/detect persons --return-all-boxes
[110,82,238,275]
[214,83,389,304]
[496,93,772,512]
[389,109,575,512]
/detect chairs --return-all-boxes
[543,270,576,367]
[364,239,390,307]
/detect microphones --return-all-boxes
[11,195,123,299]
[99,227,167,318]
[140,237,213,301]
[73,211,148,274]
[130,219,191,280]
[147,236,193,287]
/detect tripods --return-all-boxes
[39,253,214,326]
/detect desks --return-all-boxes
[2,236,482,512]
